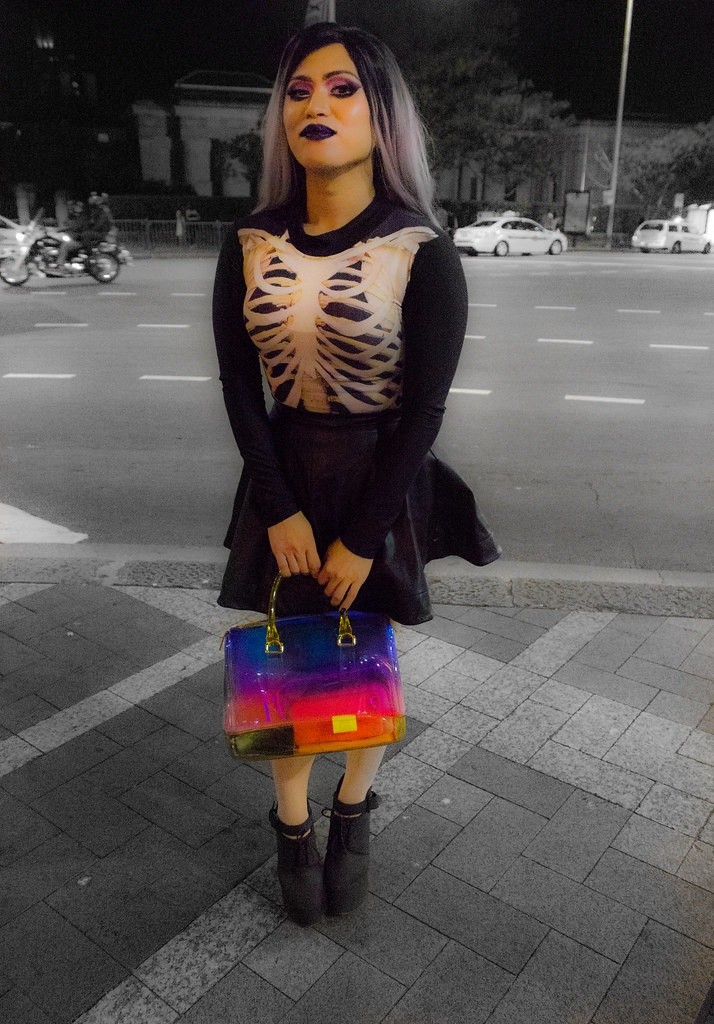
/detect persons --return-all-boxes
[45,192,115,273]
[212,22,501,927]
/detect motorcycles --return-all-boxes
[0,213,134,286]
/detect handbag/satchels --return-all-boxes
[220,567,408,762]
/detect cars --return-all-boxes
[453,217,568,257]
[630,220,711,255]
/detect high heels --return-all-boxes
[269,774,383,929]
